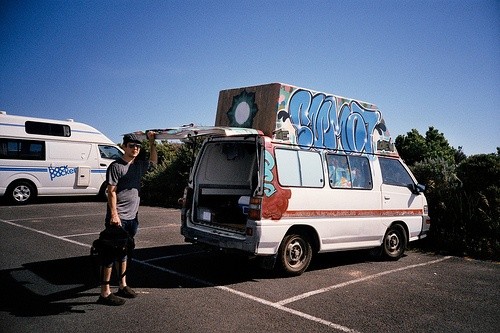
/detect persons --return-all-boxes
[97,130,158,305]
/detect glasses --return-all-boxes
[125,144,140,148]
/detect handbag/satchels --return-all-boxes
[92,223,135,267]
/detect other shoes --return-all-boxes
[118,286,136,298]
[99,293,126,306]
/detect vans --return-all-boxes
[0,111,125,205]
[134,123,432,278]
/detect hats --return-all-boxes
[123,133,142,144]
